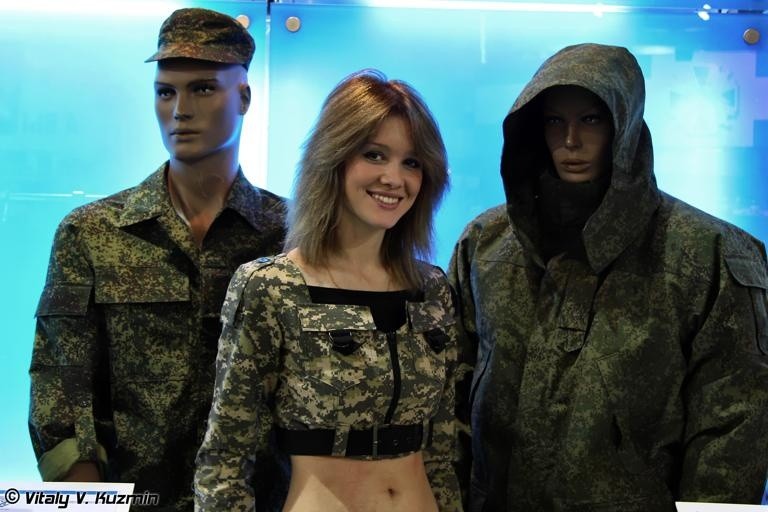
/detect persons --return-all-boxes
[26,7,297,512]
[445,42,768,510]
[191,66,462,511]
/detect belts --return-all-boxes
[276,418,425,459]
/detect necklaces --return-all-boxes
[321,259,394,293]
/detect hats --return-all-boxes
[145,7,259,73]
